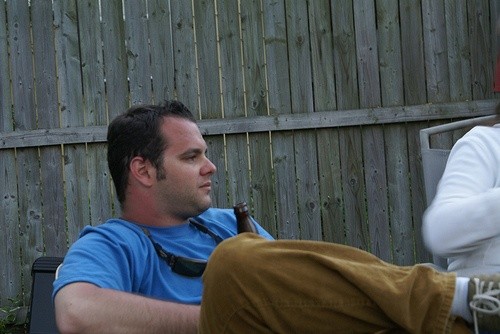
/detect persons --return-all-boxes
[421,57,500,280]
[48,100,500,334]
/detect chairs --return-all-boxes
[28,256,64,334]
[418,113,500,270]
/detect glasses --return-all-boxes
[143,220,224,277]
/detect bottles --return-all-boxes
[232,201,256,237]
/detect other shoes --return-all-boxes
[467,271,499,333]
